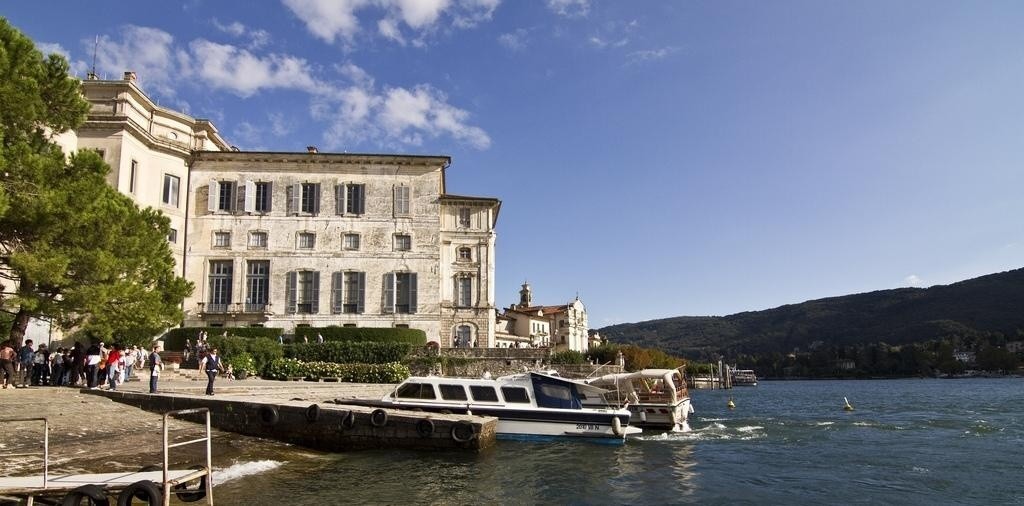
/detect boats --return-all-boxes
[496,365,695,431]
[730,368,758,386]
[329,371,642,449]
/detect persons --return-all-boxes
[304,335,308,343]
[0,339,148,391]
[278,335,283,345]
[454,336,477,350]
[183,330,233,396]
[149,345,163,394]
[316,332,323,343]
[482,368,492,380]
[495,339,538,349]
